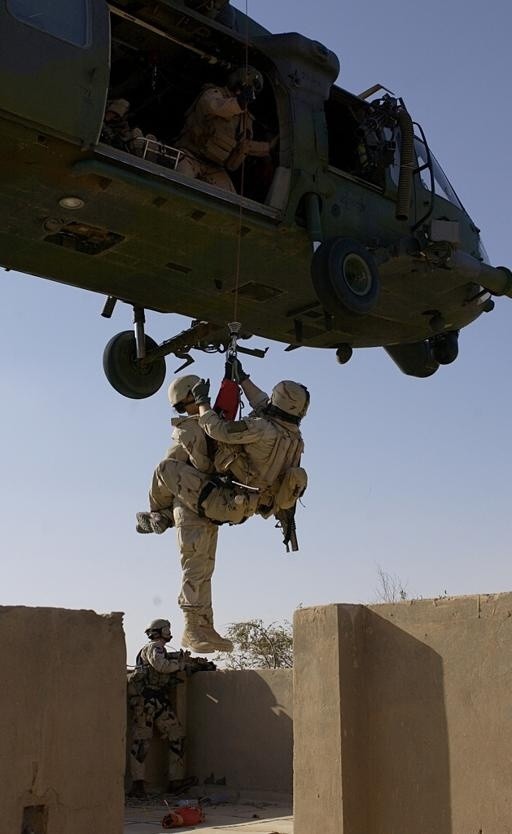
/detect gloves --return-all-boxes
[192,378,211,406]
[226,357,250,385]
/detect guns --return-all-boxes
[184,454,261,496]
[164,649,217,673]
[276,507,299,553]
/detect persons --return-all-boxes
[125,617,193,799]
[167,371,249,656]
[135,375,314,537]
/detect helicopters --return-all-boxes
[1,0,511,402]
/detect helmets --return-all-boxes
[146,619,173,643]
[168,375,200,408]
[272,380,310,417]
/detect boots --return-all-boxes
[126,780,146,798]
[182,623,233,653]
[135,511,173,534]
[169,777,198,796]
